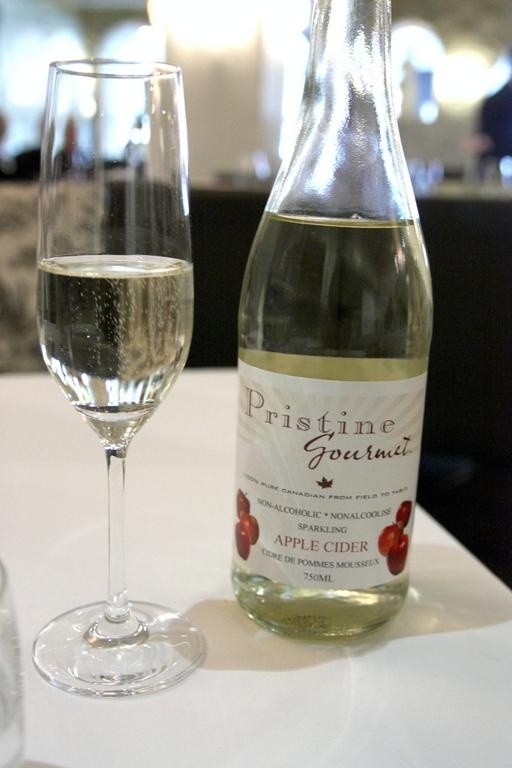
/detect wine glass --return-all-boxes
[33,58,208,700]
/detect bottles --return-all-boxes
[229,0,439,641]
